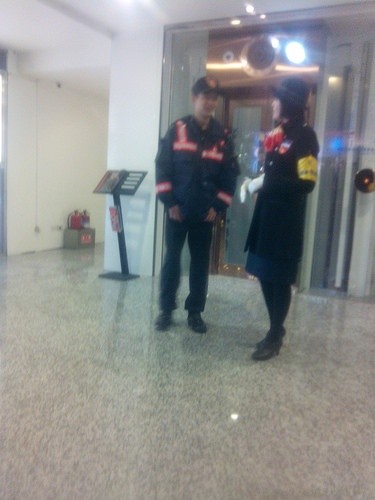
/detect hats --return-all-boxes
[271,75,309,111]
[192,76,219,93]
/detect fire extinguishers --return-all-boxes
[67,210,90,229]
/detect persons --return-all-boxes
[239,75,320,359]
[154,75,240,332]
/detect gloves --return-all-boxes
[248,174,265,194]
[240,177,252,203]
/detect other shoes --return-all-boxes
[187,313,207,333]
[154,312,171,331]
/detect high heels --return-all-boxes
[251,326,286,360]
